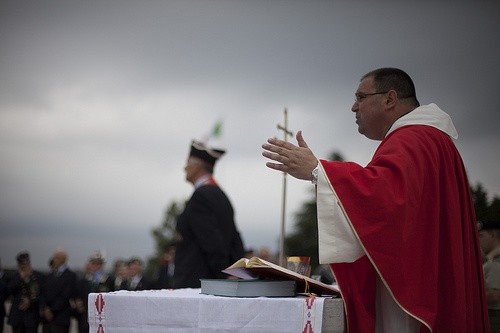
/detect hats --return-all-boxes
[190,140,225,166]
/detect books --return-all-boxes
[220,257,342,296]
[199,277,295,298]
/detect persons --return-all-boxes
[172,140,247,289]
[477,219,500,333]
[263,59,490,333]
[0,248,274,333]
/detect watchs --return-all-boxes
[312,163,318,184]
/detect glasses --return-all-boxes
[355,90,398,102]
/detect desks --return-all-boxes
[88,288,345,333]
